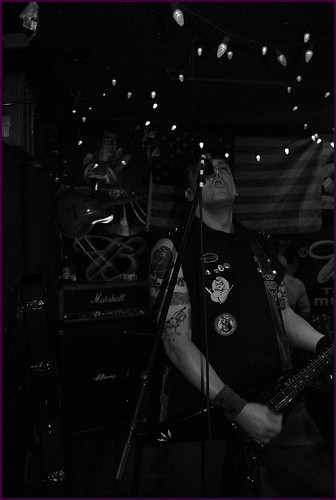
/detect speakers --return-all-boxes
[54,277,162,437]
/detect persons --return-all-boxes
[148,153,334,498]
[83,129,144,238]
[277,255,311,321]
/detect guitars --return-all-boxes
[217,336,336,473]
[51,186,148,238]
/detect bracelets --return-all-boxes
[315,335,332,353]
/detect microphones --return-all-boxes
[204,158,218,181]
